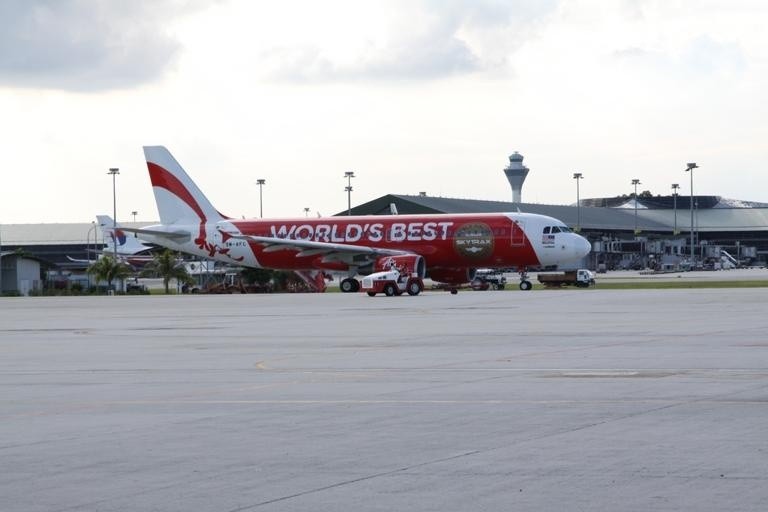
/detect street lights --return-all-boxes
[685,162,700,258]
[670,184,681,238]
[630,178,642,233]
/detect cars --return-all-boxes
[470,268,507,291]
[361,268,425,296]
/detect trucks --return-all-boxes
[537,269,594,288]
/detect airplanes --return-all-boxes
[94,145,592,296]
